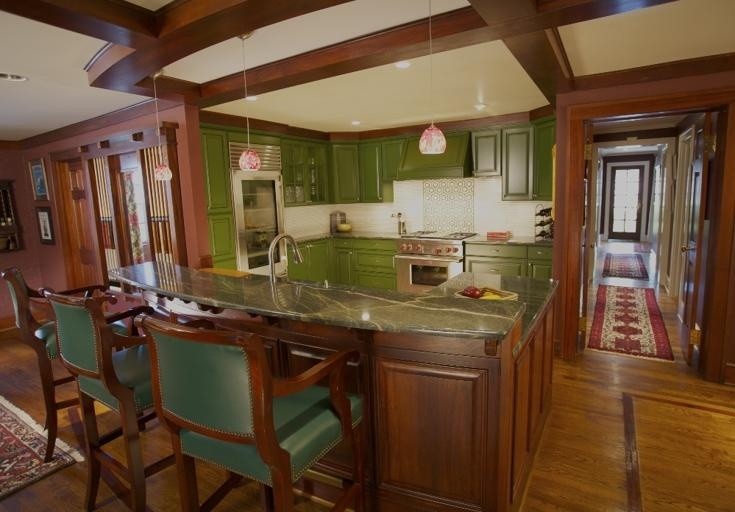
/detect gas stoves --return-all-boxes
[401,230,478,240]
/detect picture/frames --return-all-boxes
[27,157,50,202]
[34,206,54,244]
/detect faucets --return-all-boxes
[270,278,303,313]
[268,233,303,278]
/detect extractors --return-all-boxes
[397,131,473,181]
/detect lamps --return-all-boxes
[148,69,172,182]
[418,0,448,157]
[236,33,262,172]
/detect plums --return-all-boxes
[464,287,481,298]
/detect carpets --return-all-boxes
[588,281,676,363]
[602,238,653,279]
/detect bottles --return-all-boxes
[392,213,407,234]
[284,182,304,202]
[330,210,346,234]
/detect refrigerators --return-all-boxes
[230,169,288,276]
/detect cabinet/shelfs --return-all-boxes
[201,129,232,212]
[209,212,237,260]
[330,142,394,202]
[287,242,329,284]
[329,239,353,286]
[351,250,396,291]
[470,127,502,177]
[527,247,552,281]
[281,138,330,206]
[463,245,529,278]
[502,123,555,200]
[378,139,402,181]
[0,177,22,254]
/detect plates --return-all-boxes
[487,229,511,239]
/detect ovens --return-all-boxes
[394,254,464,296]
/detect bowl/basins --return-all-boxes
[337,224,352,232]
